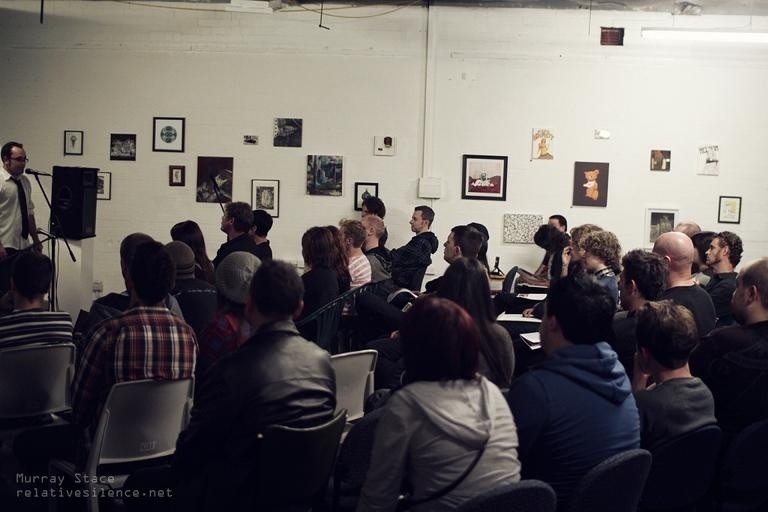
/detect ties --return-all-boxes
[10,177,29,240]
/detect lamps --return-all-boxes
[636,8,767,49]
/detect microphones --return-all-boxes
[35,227,56,240]
[25,168,50,177]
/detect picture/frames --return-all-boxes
[641,205,681,251]
[96,170,114,201]
[715,194,743,226]
[250,177,281,220]
[61,128,86,157]
[460,152,510,202]
[352,180,380,211]
[151,115,186,155]
[166,162,186,187]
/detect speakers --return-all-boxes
[49,165,100,240]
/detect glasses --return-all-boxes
[8,157,29,163]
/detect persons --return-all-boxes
[297,224,341,355]
[704,231,743,327]
[632,304,717,449]
[61,241,199,472]
[612,246,671,380]
[390,204,440,294]
[522,222,607,320]
[328,225,353,355]
[336,219,374,317]
[172,259,337,512]
[650,231,717,331]
[211,202,259,269]
[251,211,278,260]
[374,256,516,397]
[0,249,76,475]
[534,215,570,279]
[359,295,522,508]
[71,231,187,360]
[672,216,704,238]
[466,222,498,280]
[504,272,640,485]
[506,226,572,286]
[688,255,767,439]
[427,223,496,321]
[194,250,264,382]
[688,233,718,263]
[168,220,218,293]
[355,195,387,224]
[575,232,623,299]
[356,215,396,284]
[1,141,45,299]
[535,138,552,160]
[159,241,218,316]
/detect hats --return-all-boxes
[164,241,196,286]
[214,251,262,304]
